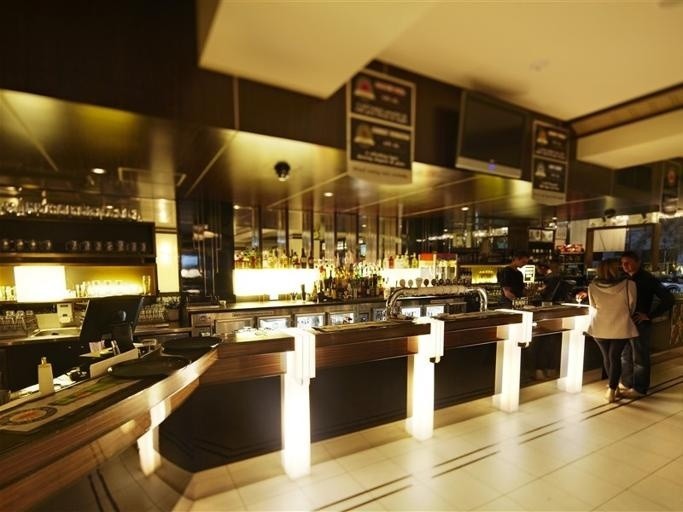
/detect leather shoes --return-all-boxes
[606,382,645,403]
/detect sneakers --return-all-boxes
[546,369,559,378]
[535,369,553,381]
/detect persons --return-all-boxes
[573,251,676,398]
[535,260,567,305]
[586,258,638,403]
[496,249,530,312]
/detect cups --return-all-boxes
[0,200,139,223]
[219,300,226,310]
[0,237,148,254]
[0,389,10,405]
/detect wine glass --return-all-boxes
[575,294,582,309]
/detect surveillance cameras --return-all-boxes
[275,162,291,181]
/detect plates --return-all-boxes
[104,355,192,380]
[161,336,223,351]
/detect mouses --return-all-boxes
[69,372,79,380]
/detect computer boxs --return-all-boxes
[78,344,154,372]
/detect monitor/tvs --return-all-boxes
[551,280,577,302]
[80,295,145,350]
[455,89,529,179]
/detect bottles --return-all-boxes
[529,248,552,263]
[458,267,471,285]
[427,308,439,316]
[385,250,417,269]
[0,274,182,340]
[288,262,385,302]
[403,310,419,316]
[36,355,54,397]
[295,316,319,327]
[511,297,527,310]
[233,246,339,268]
[477,265,494,278]
[475,280,543,304]
